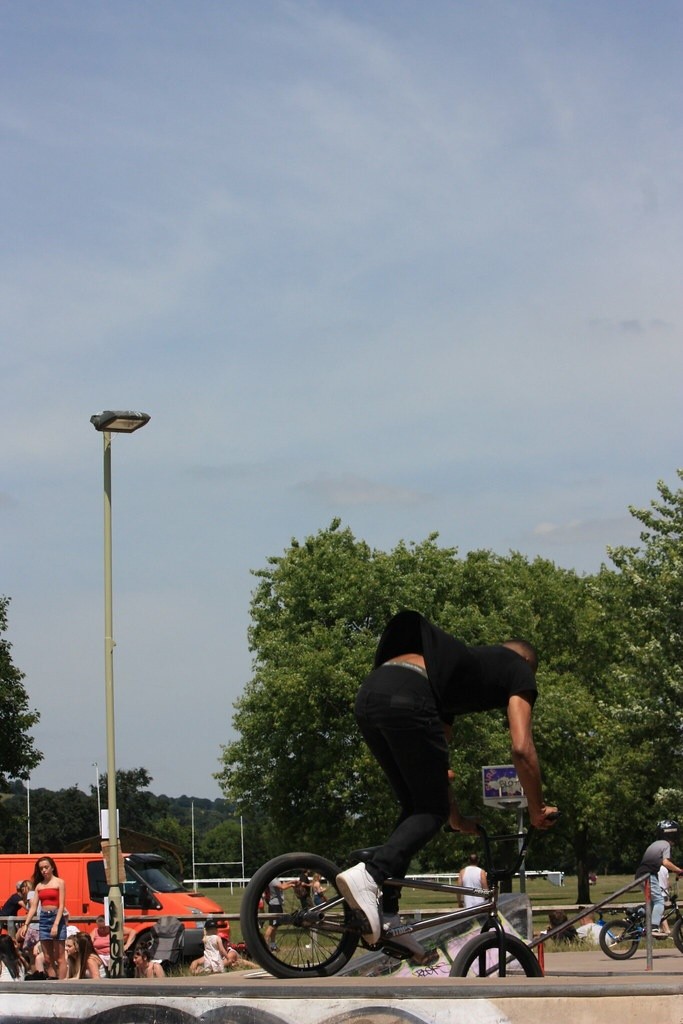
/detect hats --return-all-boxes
[16,881,23,889]
[204,920,218,929]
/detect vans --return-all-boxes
[1,853,231,966]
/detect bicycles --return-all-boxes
[239,811,544,978]
[598,870,683,961]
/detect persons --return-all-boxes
[635,835,683,938]
[576,909,611,945]
[456,855,490,909]
[131,947,166,977]
[0,880,26,934]
[0,909,108,981]
[542,910,578,943]
[20,856,67,979]
[202,920,228,974]
[336,609,557,945]
[189,934,241,974]
[589,871,597,882]
[20,880,41,949]
[89,915,136,970]
[259,869,331,953]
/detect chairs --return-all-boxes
[126,916,184,969]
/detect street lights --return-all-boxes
[90,408,152,978]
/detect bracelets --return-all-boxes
[25,924,28,926]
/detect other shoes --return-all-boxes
[271,946,281,952]
[651,928,668,936]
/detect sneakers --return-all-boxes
[337,862,383,944]
[379,915,424,955]
